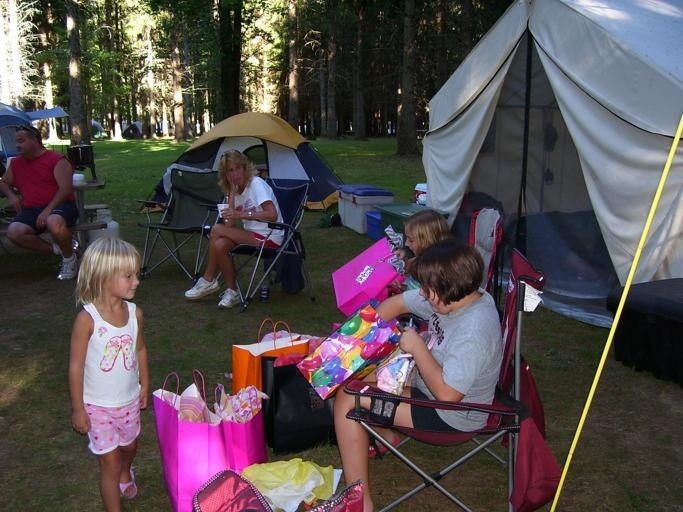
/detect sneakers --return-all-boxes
[218,287,242,309]
[57,253,78,281]
[184,277,220,300]
[52,239,79,255]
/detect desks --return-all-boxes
[0,178,106,254]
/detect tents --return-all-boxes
[124,121,142,139]
[91,119,108,139]
[0,102,69,197]
[422,0,682,329]
[140,112,345,215]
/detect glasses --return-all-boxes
[15,124,37,136]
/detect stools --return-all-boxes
[605,278,682,386]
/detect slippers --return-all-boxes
[119,469,138,500]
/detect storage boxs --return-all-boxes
[336,183,395,234]
[372,201,450,248]
[364,210,383,240]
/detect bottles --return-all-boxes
[260,287,269,302]
[402,317,420,331]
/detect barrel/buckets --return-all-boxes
[415,184,427,206]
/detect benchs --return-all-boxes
[84,203,109,211]
[1,221,106,236]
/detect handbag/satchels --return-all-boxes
[231,320,338,455]
[294,300,407,401]
[153,369,267,511]
[332,235,405,317]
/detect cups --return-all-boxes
[218,204,230,219]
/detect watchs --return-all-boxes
[248,212,252,220]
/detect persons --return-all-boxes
[388,210,454,297]
[185,150,285,309]
[333,243,503,512]
[68,237,150,512]
[0,126,79,281]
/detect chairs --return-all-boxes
[206,173,318,313]
[135,164,225,282]
[387,190,506,334]
[342,251,562,512]
